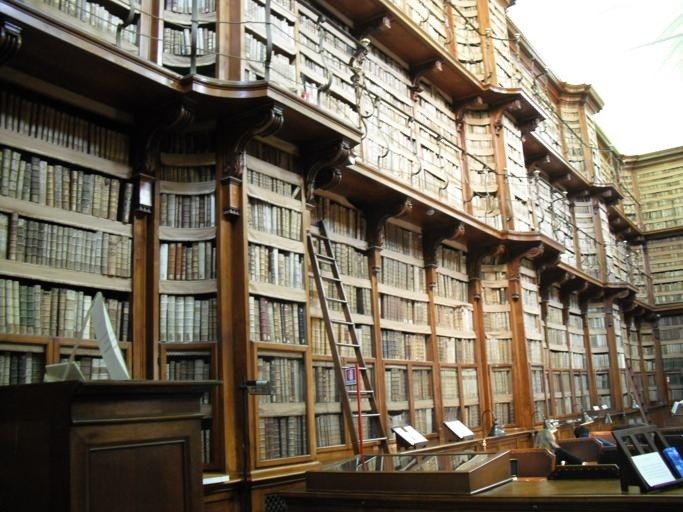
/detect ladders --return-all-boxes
[308,219,390,456]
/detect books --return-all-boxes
[1,0,681,485]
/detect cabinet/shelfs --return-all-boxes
[1,0,683,512]
